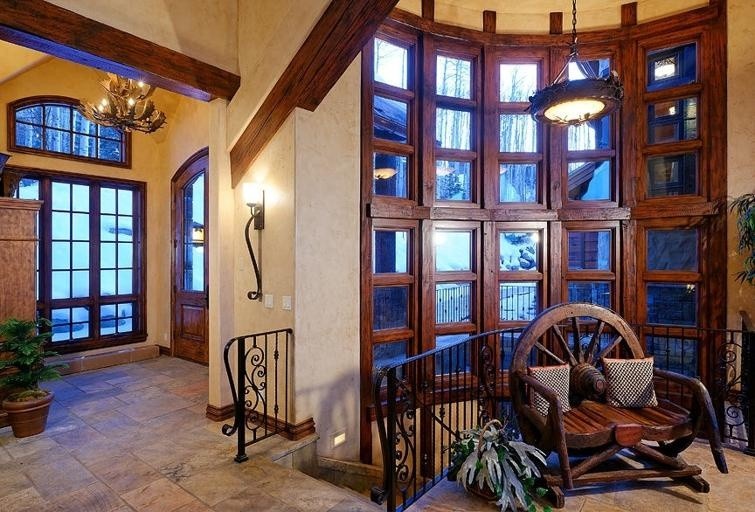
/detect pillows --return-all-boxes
[524,362,571,418]
[603,355,660,410]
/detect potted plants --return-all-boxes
[0,316,70,438]
[446,418,556,510]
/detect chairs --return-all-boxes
[509,299,731,511]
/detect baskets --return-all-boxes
[465,420,503,500]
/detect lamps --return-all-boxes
[75,72,169,136]
[191,221,204,248]
[240,182,267,302]
[525,0,627,130]
